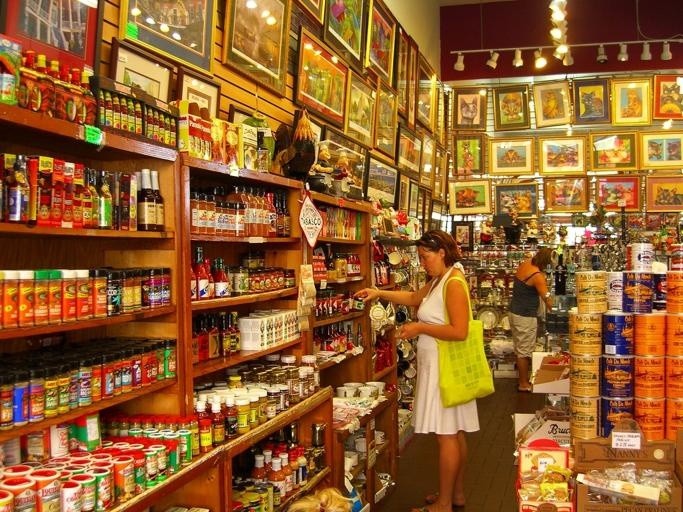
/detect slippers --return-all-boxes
[427,492,465,509]
[413,507,428,512]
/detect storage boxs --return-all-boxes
[517,447,568,478]
[576,472,683,512]
[516,487,574,512]
[574,437,675,474]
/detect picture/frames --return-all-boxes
[449,74,683,214]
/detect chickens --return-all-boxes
[272,103,316,180]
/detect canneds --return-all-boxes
[314,425,323,446]
[567,242,683,441]
[275,429,285,441]
[284,423,298,441]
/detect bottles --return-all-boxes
[0,266,171,329]
[187,246,231,301]
[193,311,241,366]
[0,335,176,431]
[195,395,238,447]
[247,443,308,496]
[21,50,97,127]
[313,322,363,353]
[0,154,165,231]
[190,183,291,238]
[100,88,178,148]
[108,414,199,456]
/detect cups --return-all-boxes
[354,438,367,452]
[396,337,417,402]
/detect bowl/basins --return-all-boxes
[336,382,386,401]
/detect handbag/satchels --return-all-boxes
[433,320,495,407]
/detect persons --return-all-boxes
[354,229,481,512]
[507,247,553,391]
